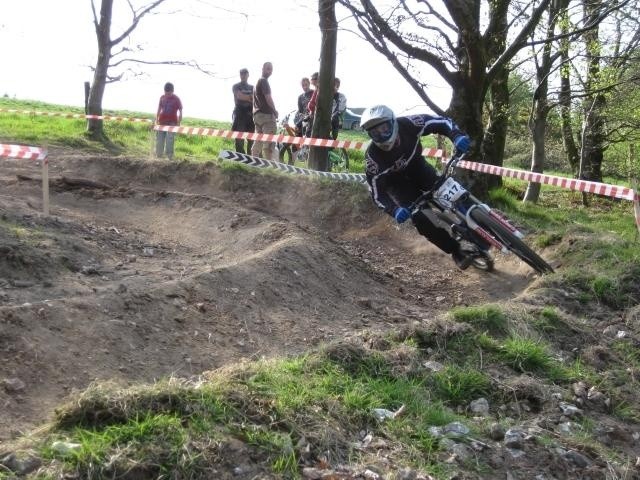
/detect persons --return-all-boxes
[358,104,472,271]
[250,61,278,160]
[230,68,255,154]
[293,71,347,162]
[154,81,183,160]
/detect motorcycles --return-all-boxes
[399,147,561,283]
[271,107,353,174]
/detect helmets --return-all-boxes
[359,105,399,151]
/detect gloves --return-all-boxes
[454,134,471,153]
[394,206,410,224]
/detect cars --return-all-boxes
[338,106,367,132]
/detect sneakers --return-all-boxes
[452,249,472,270]
